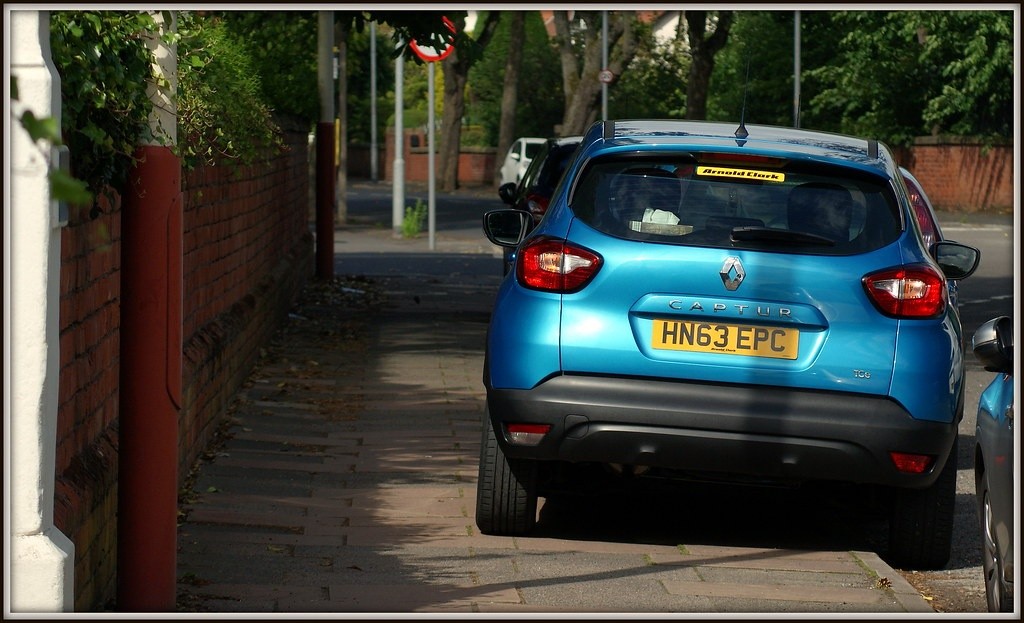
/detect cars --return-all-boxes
[499,137,960,319]
[969,318,1014,611]
[475,120,981,567]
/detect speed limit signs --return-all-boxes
[600,71,615,82]
